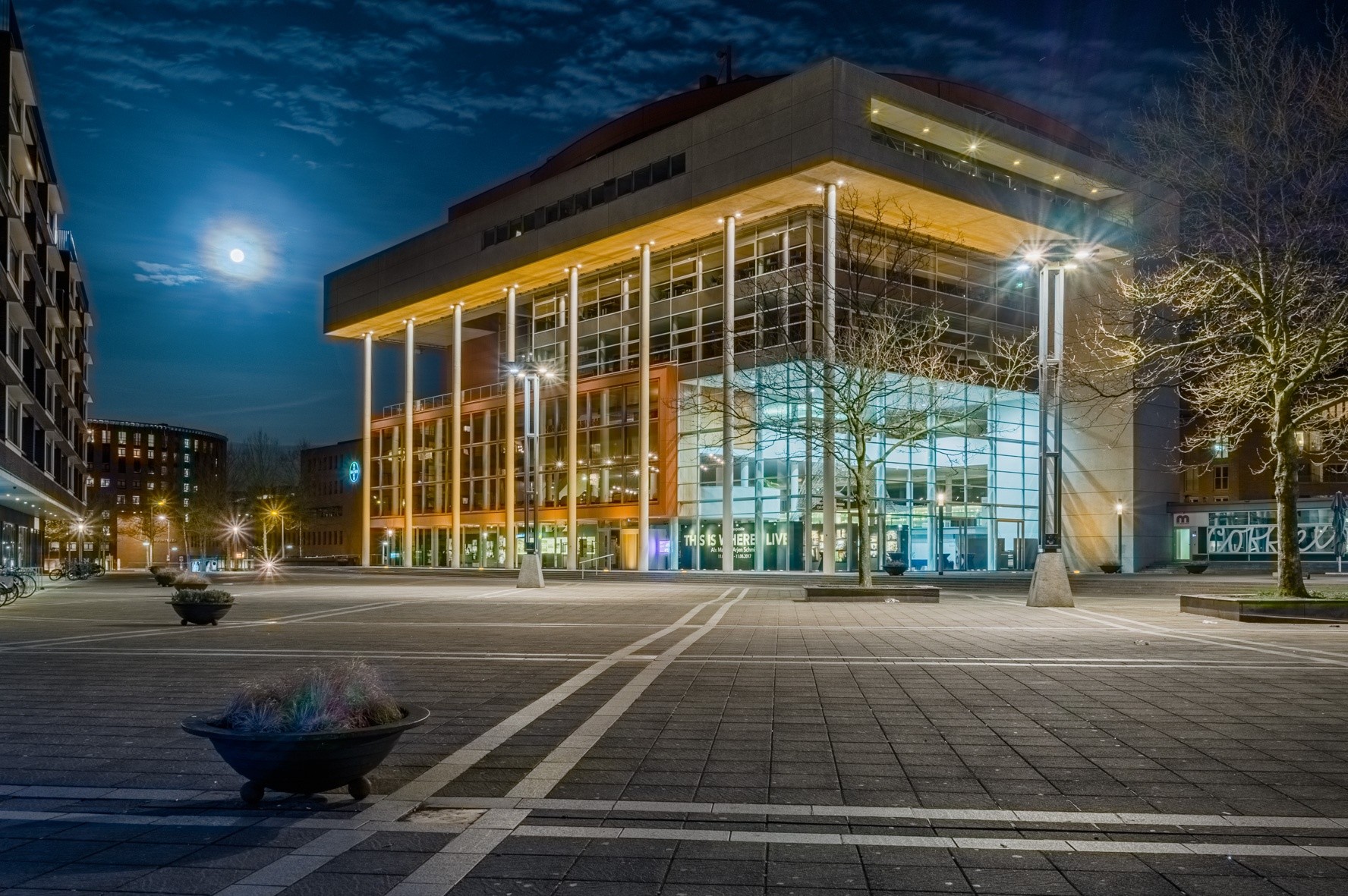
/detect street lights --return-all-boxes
[232,526,239,571]
[78,524,86,560]
[1007,237,1097,608]
[509,362,557,589]
[936,493,946,575]
[272,512,284,561]
[1115,499,1124,573]
[158,515,170,567]
[151,498,166,565]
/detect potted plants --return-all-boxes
[165,588,239,626]
[880,561,910,576]
[180,652,432,804]
[146,565,210,593]
[1184,561,1209,574]
[1097,561,1123,574]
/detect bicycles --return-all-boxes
[49,561,106,581]
[0,565,37,607]
